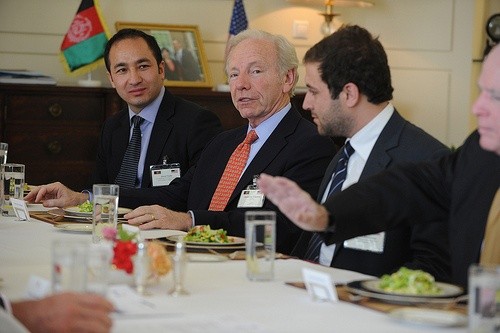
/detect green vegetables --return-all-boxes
[184,224,235,244]
[380,266,443,295]
[79,200,94,213]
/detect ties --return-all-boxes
[305,140,355,265]
[112,115,145,189]
[207,129,259,211]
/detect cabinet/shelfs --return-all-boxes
[0,87,314,195]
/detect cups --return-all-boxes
[0,142,25,216]
[245,211,276,281]
[50,237,115,298]
[467,265,500,333]
[92,184,119,240]
[137,234,187,295]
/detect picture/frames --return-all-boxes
[114,20,215,88]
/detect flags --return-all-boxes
[61,0,112,78]
[227,0,248,38]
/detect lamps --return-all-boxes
[287,0,375,37]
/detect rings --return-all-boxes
[149,214,156,220]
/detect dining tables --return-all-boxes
[1,205,471,333]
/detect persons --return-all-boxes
[25,30,338,258]
[0,291,115,333]
[69,29,223,194]
[258,42,500,311]
[162,48,180,80]
[287,26,455,282]
[172,40,200,81]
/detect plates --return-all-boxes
[151,232,265,250]
[47,205,132,223]
[342,277,469,305]
